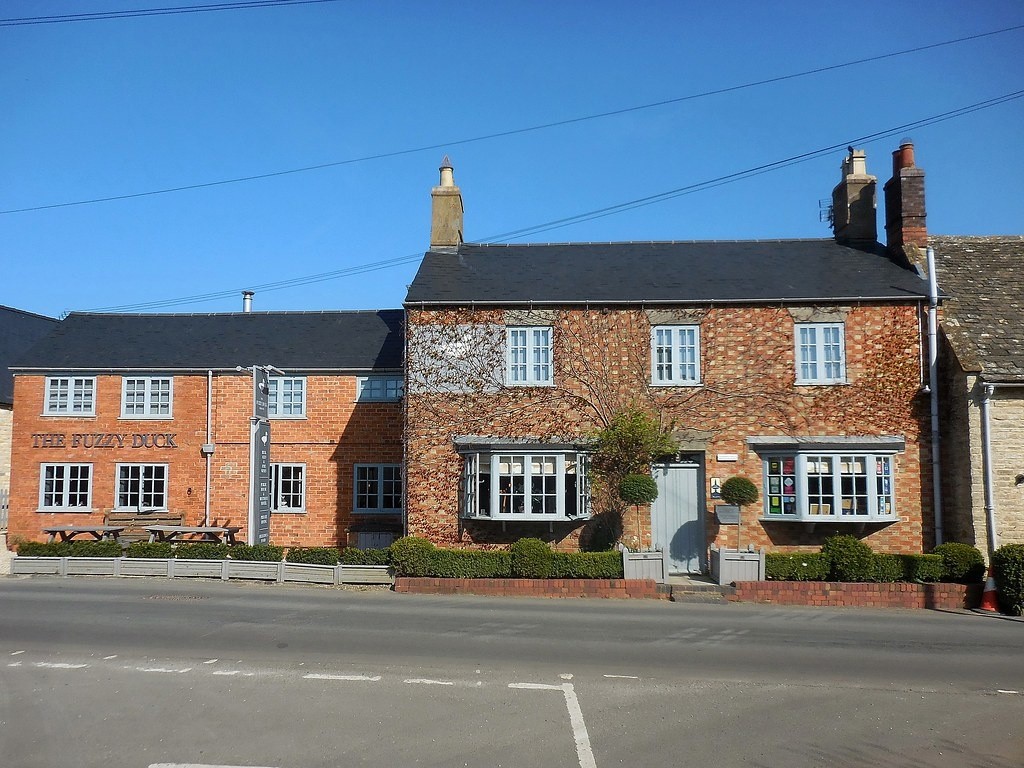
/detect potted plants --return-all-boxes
[708,542,766,586]
[619,543,670,585]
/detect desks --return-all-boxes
[41,526,124,545]
[142,523,243,545]
